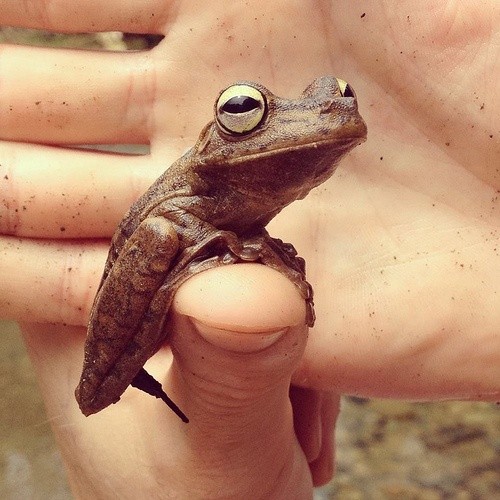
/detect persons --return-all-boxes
[0,1,500,500]
